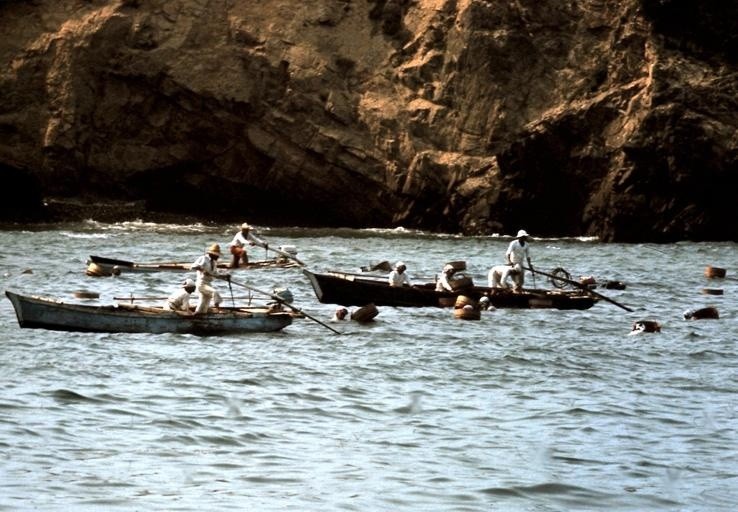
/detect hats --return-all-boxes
[514,229,530,238]
[236,221,255,231]
[206,243,225,257]
[180,278,196,288]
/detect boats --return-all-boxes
[5,290,293,336]
[302,268,601,311]
[90,255,298,272]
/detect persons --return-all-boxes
[190,244,231,318]
[162,278,196,316]
[228,222,269,268]
[388,261,421,290]
[505,229,535,294]
[435,265,456,295]
[488,262,525,294]
